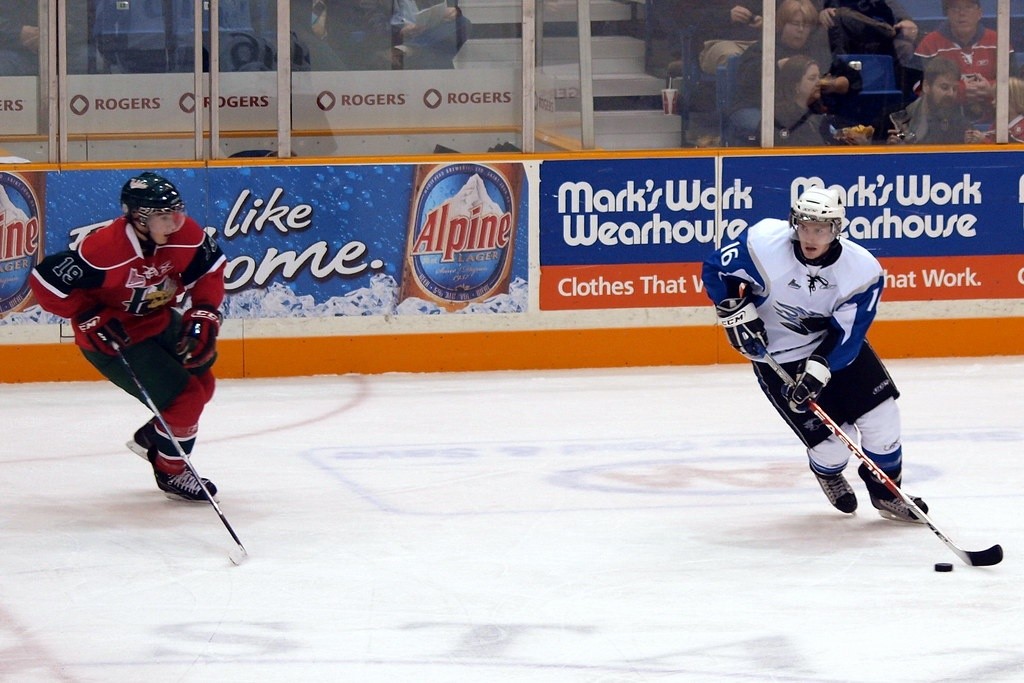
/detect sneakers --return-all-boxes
[126,420,156,461]
[155,453,218,503]
[816,474,858,518]
[871,493,928,523]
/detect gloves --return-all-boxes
[781,354,831,414]
[716,296,769,359]
[77,305,129,355]
[177,305,223,371]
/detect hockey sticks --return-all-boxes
[757,343,1003,568]
[113,343,249,566]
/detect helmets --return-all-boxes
[120,172,186,227]
[789,185,843,236]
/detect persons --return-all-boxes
[30,173,227,504]
[0,0,1024,146]
[886,57,974,144]
[703,187,928,524]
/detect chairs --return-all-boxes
[642,4,683,88]
[836,54,902,145]
[717,54,740,147]
[91,0,270,76]
[682,25,716,147]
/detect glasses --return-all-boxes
[947,2,979,14]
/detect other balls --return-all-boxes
[935,563,953,572]
